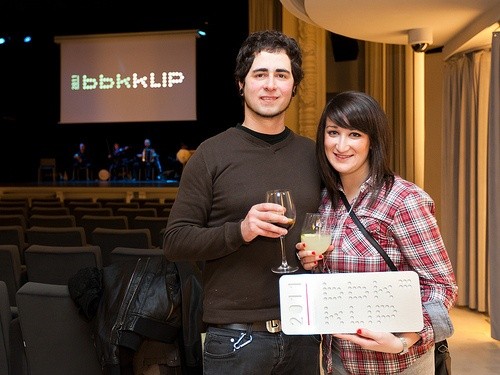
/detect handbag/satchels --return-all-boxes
[433,338,453,375]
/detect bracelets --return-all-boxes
[395,334,408,355]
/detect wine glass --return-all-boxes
[266,187,299,274]
[301,213,332,274]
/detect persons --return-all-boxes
[108,143,123,162]
[163,29,327,375]
[142,139,154,163]
[295,91,460,375]
[74,143,90,165]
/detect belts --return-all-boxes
[215,320,282,333]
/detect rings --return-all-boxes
[296,252,303,261]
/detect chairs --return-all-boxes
[0,198,203,375]
